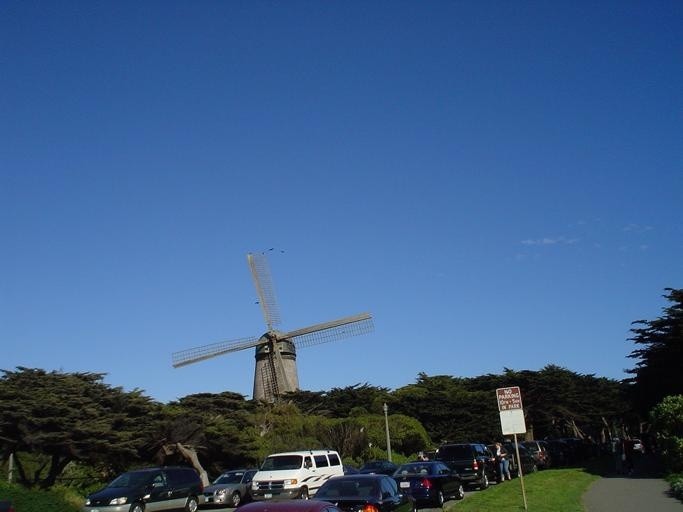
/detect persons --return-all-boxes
[491,442,511,483]
[615,438,634,476]
[417,452,430,461]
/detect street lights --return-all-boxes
[380,403,392,461]
